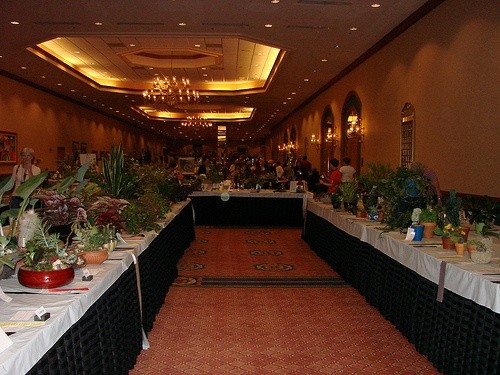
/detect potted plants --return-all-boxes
[32,186,88,247]
[0,235,19,280]
[331,161,492,263]
[199,171,286,193]
[102,224,118,253]
[18,219,85,289]
[71,221,109,265]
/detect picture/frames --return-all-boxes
[71,142,109,163]
[0,131,18,165]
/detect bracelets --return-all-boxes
[437,198,441,199]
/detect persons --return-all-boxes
[2,147,41,225]
[352,177,366,198]
[32,149,320,190]
[409,161,442,207]
[321,158,343,196]
[339,157,356,185]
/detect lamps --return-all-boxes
[218,126,226,141]
[181,116,212,127]
[143,75,200,102]
[278,110,365,157]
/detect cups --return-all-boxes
[412,225,423,241]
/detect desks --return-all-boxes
[187,188,314,229]
[302,198,500,375]
[0,197,196,375]
